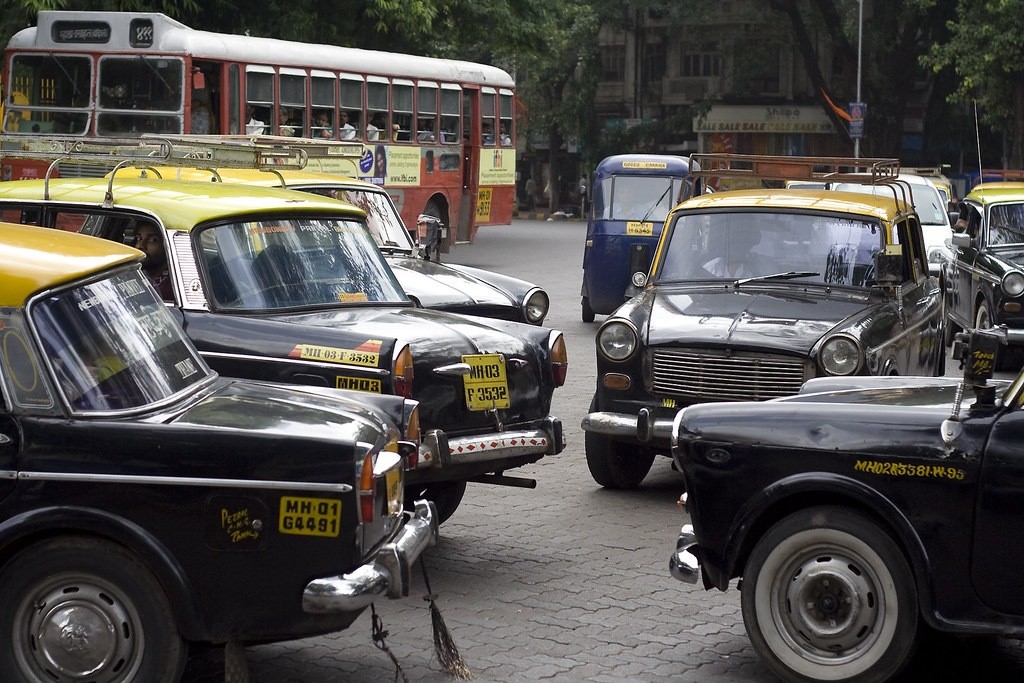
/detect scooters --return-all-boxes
[581,155,702,322]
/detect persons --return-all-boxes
[231,105,512,146]
[579,173,588,207]
[525,175,537,212]
[702,214,774,277]
[130,221,175,300]
[990,206,1021,245]
[158,79,210,134]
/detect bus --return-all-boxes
[0,10,518,253]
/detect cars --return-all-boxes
[666,323,1024,681]
[934,188,1024,367]
[0,154,568,541]
[580,154,948,491]
[784,157,961,283]
[0,223,452,683]
[82,149,554,322]
